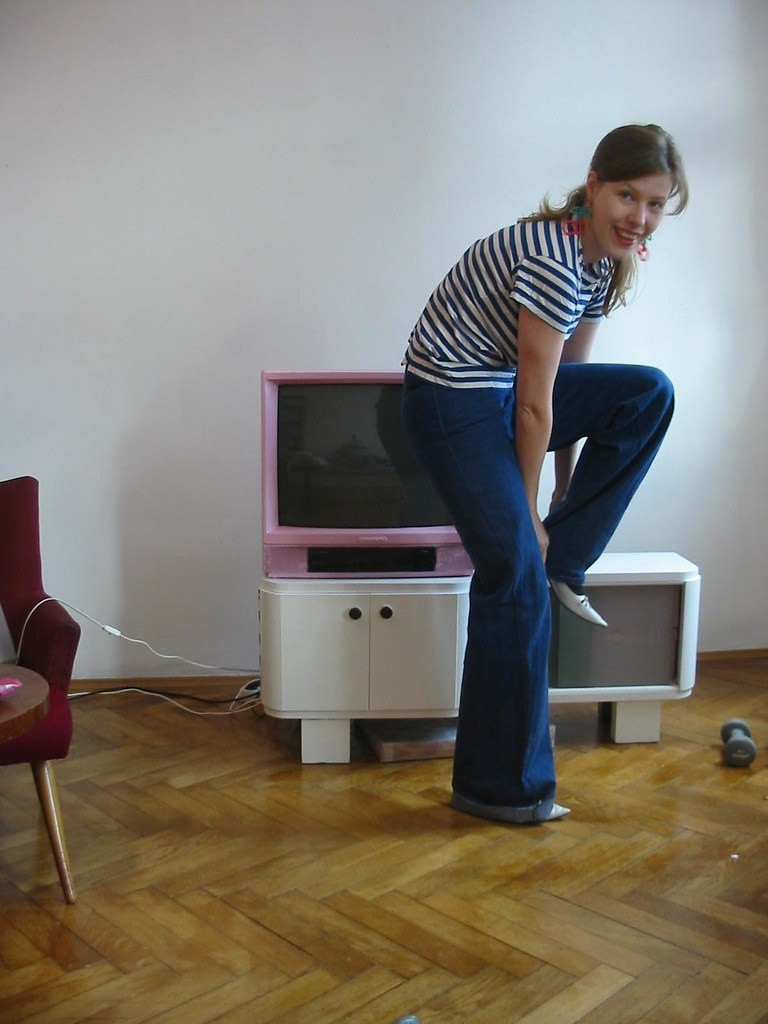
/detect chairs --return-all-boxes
[0,475,81,905]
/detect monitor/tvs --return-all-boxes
[260,371,477,576]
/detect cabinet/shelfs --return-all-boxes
[258,551,699,764]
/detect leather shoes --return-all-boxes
[548,576,609,628]
[450,790,571,823]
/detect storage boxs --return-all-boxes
[357,718,556,763]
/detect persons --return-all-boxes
[401,124,690,824]
